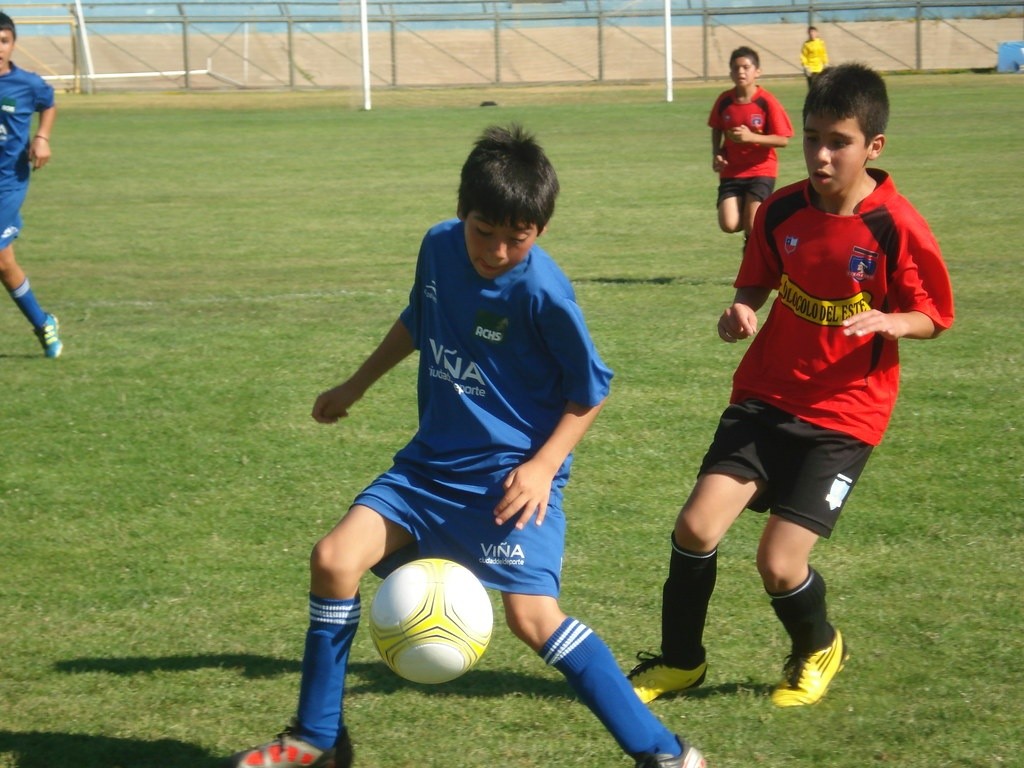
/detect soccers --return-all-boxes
[368,559,493,685]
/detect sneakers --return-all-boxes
[625,646,707,704]
[221,722,353,768]
[769,627,849,707]
[34,313,62,359]
[634,734,708,768]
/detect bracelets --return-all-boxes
[34,134,49,143]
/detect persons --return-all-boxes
[0,9,67,363]
[220,119,718,768]
[705,45,794,256]
[798,26,830,90]
[622,59,959,710]
